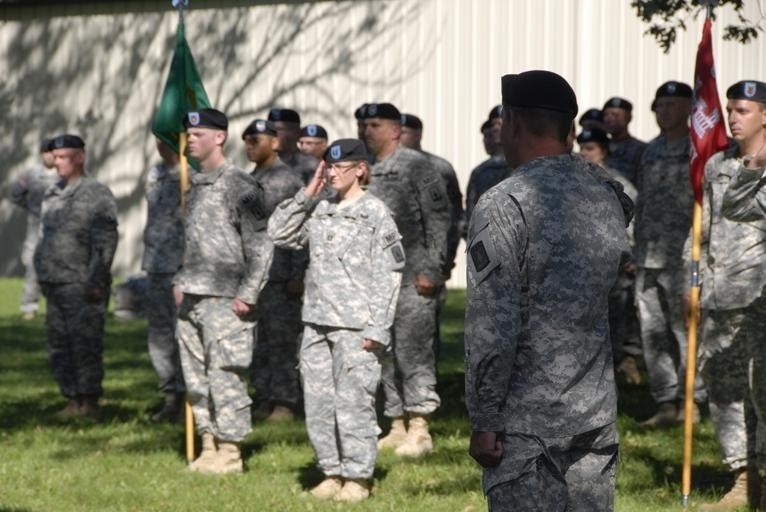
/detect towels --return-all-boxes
[39,140,49,152]
[654,81,694,99]
[299,124,328,140]
[354,102,372,121]
[268,108,301,125]
[242,118,278,140]
[488,105,503,118]
[322,138,370,164]
[726,81,766,105]
[363,103,402,121]
[181,107,228,132]
[576,126,608,143]
[48,134,86,151]
[499,71,579,119]
[603,98,634,112]
[576,109,603,126]
[401,114,423,130]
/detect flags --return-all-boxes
[152,24,213,172]
[690,19,730,207]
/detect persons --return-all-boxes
[242,119,309,424]
[299,125,328,159]
[601,98,647,183]
[579,109,605,130]
[10,140,63,320]
[462,105,513,240]
[267,139,406,505]
[142,137,197,424]
[578,128,638,248]
[172,108,274,475]
[635,81,708,427]
[362,103,453,457]
[398,113,462,283]
[464,70,632,512]
[682,80,766,512]
[33,135,118,420]
[267,110,319,188]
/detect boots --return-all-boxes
[175,431,220,473]
[150,391,186,424]
[377,416,408,451]
[332,477,371,505]
[669,400,711,429]
[304,477,343,500]
[265,407,297,426]
[634,400,681,430]
[72,396,101,429]
[391,412,436,456]
[697,466,763,512]
[250,403,270,425]
[199,442,244,477]
[50,397,79,424]
